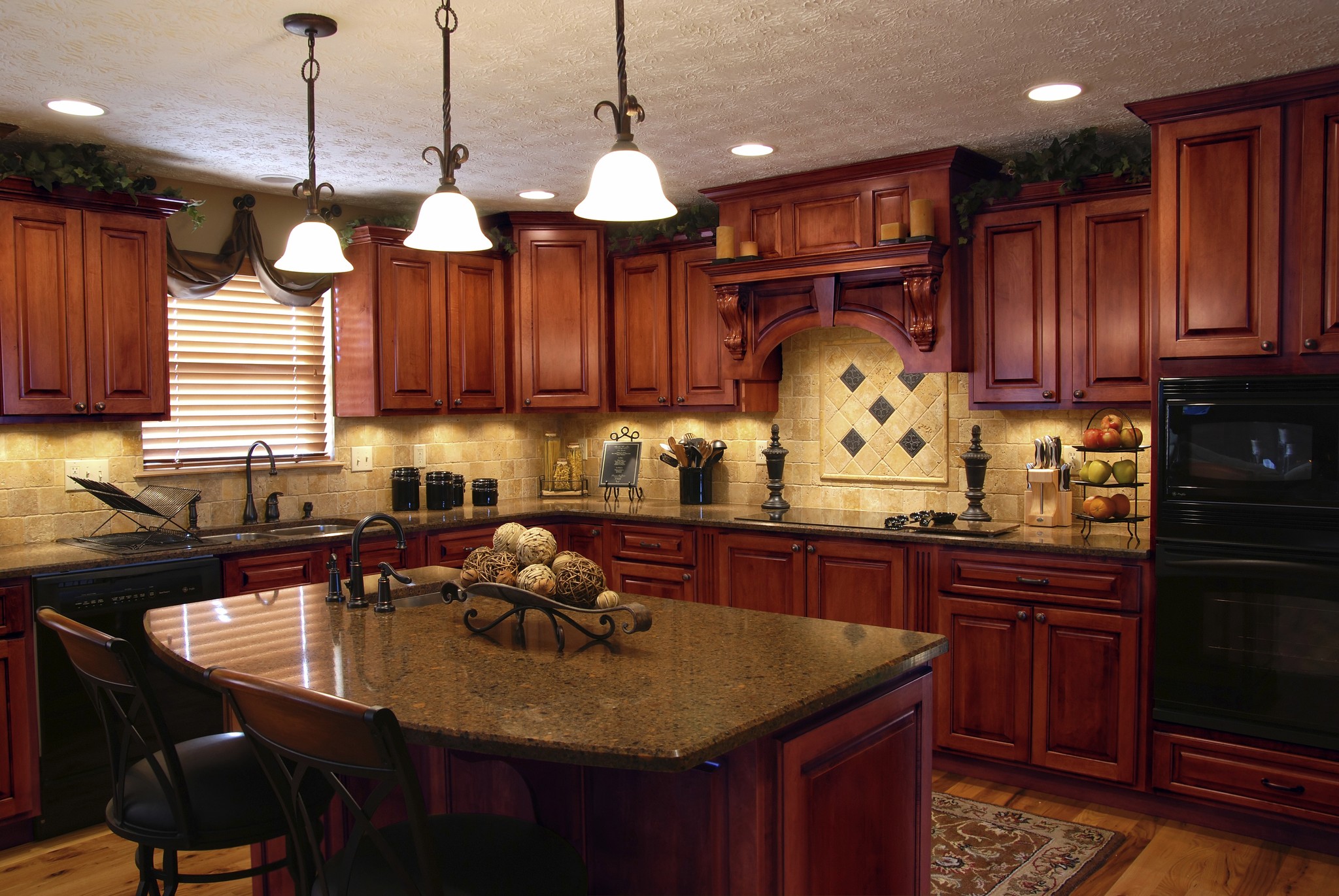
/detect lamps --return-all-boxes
[274,14,353,274]
[404,1,492,251]
[572,0,678,221]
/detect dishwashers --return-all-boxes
[33,554,225,842]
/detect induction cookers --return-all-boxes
[733,507,936,531]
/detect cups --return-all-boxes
[678,466,713,505]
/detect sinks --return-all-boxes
[364,580,488,610]
[192,530,280,549]
[266,523,369,538]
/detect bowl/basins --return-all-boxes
[930,512,958,523]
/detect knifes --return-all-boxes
[1025,436,1072,492]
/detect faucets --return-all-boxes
[344,511,409,610]
[240,440,279,527]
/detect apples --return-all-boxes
[1082,424,1103,449]
[1120,426,1143,449]
[1088,460,1113,483]
[1113,456,1136,483]
[1075,460,1094,482]
[1096,426,1121,448]
[1100,413,1123,434]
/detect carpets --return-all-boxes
[930,791,1126,895]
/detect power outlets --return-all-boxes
[413,445,426,468]
[64,460,109,492]
[755,440,768,466]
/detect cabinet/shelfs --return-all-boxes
[0,496,1339,848]
[333,62,1337,411]
[0,176,188,420]
[144,564,947,896]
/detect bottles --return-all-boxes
[544,434,583,490]
[389,466,498,511]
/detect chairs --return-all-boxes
[203,664,604,896]
[39,604,333,896]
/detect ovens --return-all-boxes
[1155,374,1339,755]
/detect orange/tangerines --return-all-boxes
[1090,496,1117,520]
[1082,493,1103,515]
[1111,494,1131,519]
